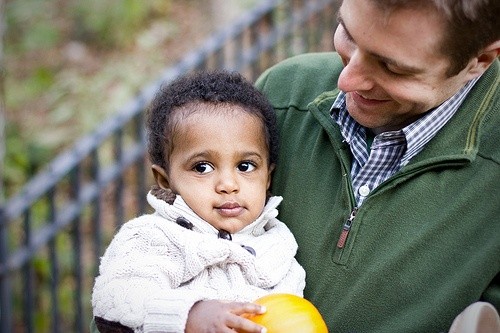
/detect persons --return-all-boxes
[92,71,306,333]
[95,0,500,333]
